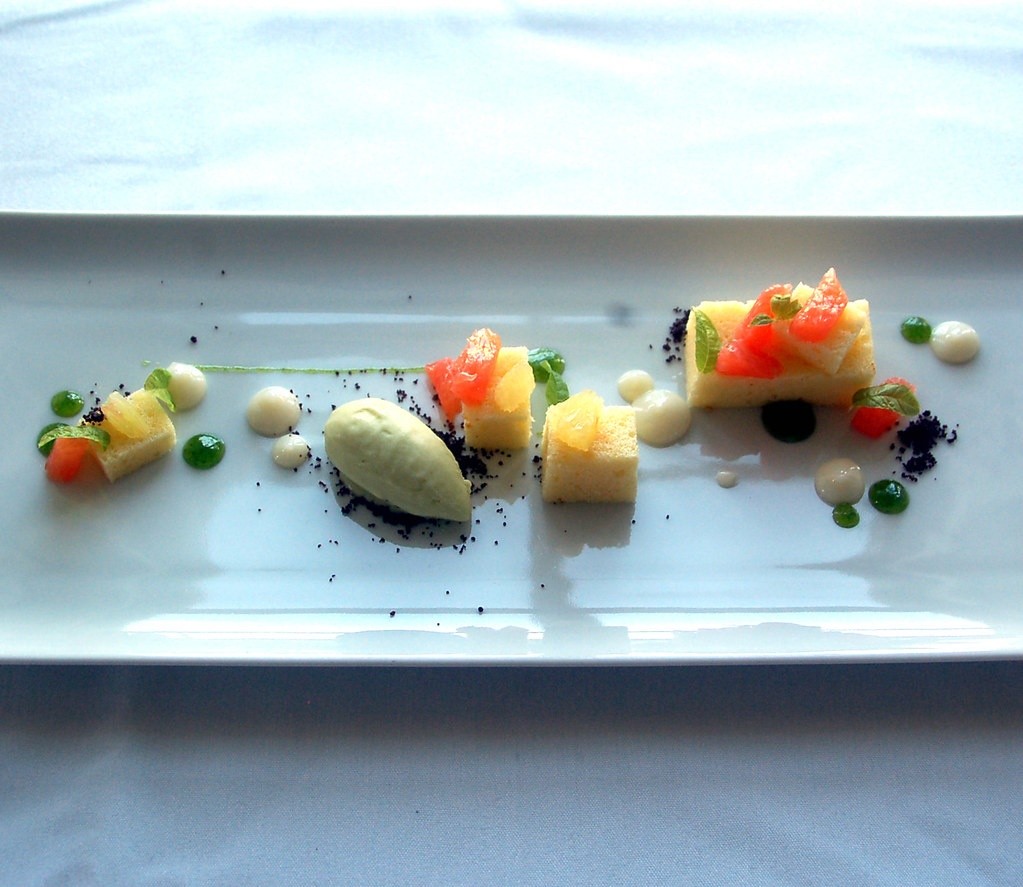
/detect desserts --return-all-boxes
[35,267,982,525]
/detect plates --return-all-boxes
[0,211,1023,668]
